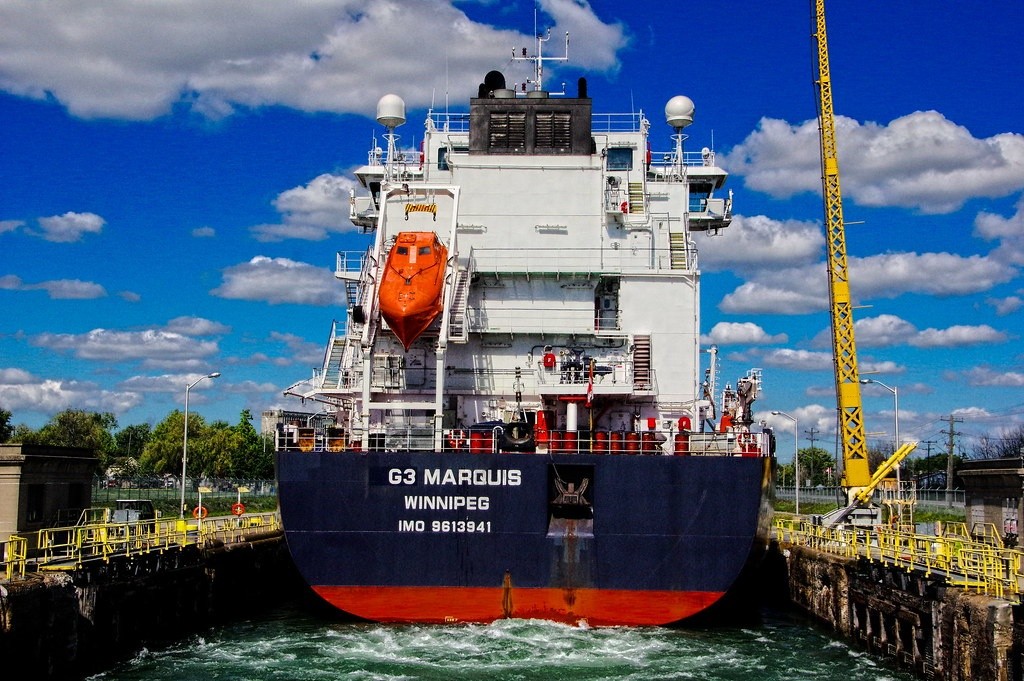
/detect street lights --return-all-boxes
[860,378,910,531]
[180,372,222,526]
[771,406,802,516]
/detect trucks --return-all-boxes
[108,498,155,535]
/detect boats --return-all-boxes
[278,9,785,628]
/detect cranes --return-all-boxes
[810,17,891,536]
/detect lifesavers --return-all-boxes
[447,429,466,446]
[738,433,755,449]
[193,506,208,520]
[621,201,627,213]
[231,503,245,515]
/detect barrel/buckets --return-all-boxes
[470,431,483,454]
[592,431,606,456]
[548,431,562,453]
[609,431,622,455]
[675,434,689,457]
[625,432,639,455]
[483,432,492,455]
[563,432,577,454]
[641,432,655,456]
